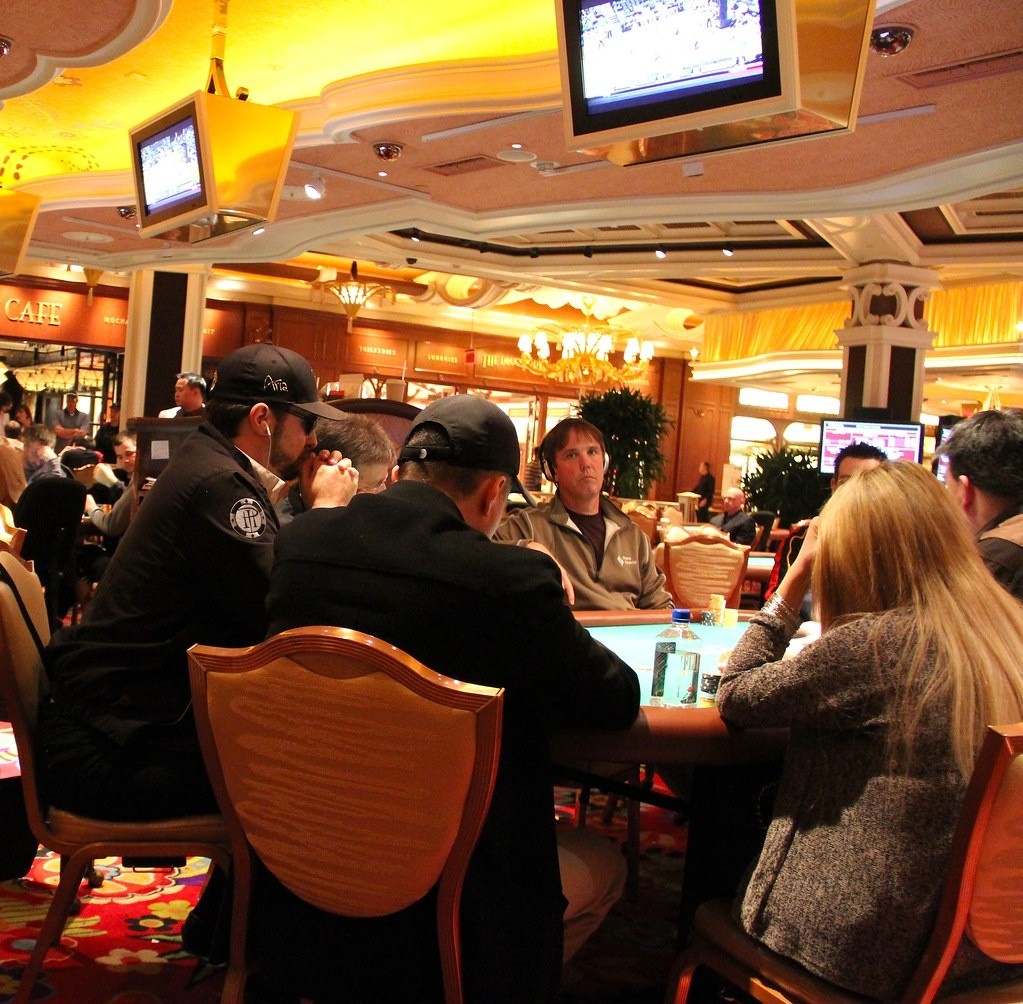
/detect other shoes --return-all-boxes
[182,911,279,968]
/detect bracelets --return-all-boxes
[760,592,803,641]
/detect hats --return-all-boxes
[210,343,349,422]
[396,394,538,509]
[110,403,121,410]
[67,390,78,399]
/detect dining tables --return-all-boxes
[567,606,822,942]
[744,550,777,610]
[765,527,792,552]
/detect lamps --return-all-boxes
[310,259,397,333]
[406,231,735,259]
[304,171,327,200]
[516,292,655,391]
[47,262,131,307]
[160,231,180,259]
[0,341,118,394]
[689,346,700,363]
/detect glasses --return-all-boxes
[273,404,319,436]
[357,472,390,494]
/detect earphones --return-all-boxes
[261,420,271,436]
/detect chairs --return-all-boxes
[0,463,1023,1004]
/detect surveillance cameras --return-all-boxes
[406,257,417,265]
[372,143,404,163]
[869,27,913,57]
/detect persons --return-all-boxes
[0,391,149,631]
[265,394,639,1004]
[645,458,1023,1004]
[938,410,1023,597]
[491,417,676,612]
[280,411,396,517]
[41,341,359,967]
[525,447,542,491]
[691,460,715,522]
[710,487,756,545]
[763,441,886,623]
[173,372,207,418]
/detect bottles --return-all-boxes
[649,610,702,707]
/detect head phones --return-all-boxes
[539,448,610,483]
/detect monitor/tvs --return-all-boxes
[817,420,925,479]
[129,90,301,252]
[554,0,877,170]
[932,426,955,487]
